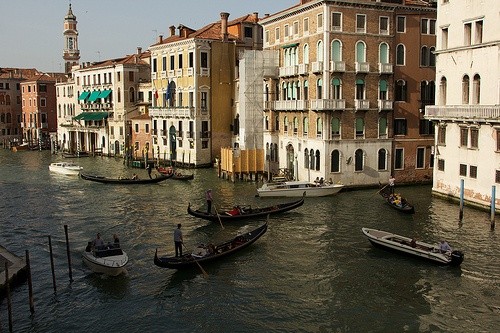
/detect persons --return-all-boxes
[146,162,152,179]
[388,176,395,195]
[85,233,120,252]
[390,193,401,206]
[439,240,453,254]
[131,173,136,180]
[173,224,184,257]
[229,205,244,216]
[315,177,324,187]
[410,237,417,248]
[207,189,214,214]
[195,242,216,257]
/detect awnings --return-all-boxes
[72,112,108,121]
[77,90,112,101]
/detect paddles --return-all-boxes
[180,241,207,278]
[151,171,157,178]
[212,201,224,230]
[369,183,389,200]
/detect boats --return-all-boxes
[79,163,195,184]
[154,214,270,269]
[254,181,345,198]
[49,162,83,176]
[362,227,464,267]
[79,236,129,277]
[187,191,307,221]
[379,180,415,214]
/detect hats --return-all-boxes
[438,238,445,241]
[208,189,212,191]
[413,238,417,241]
